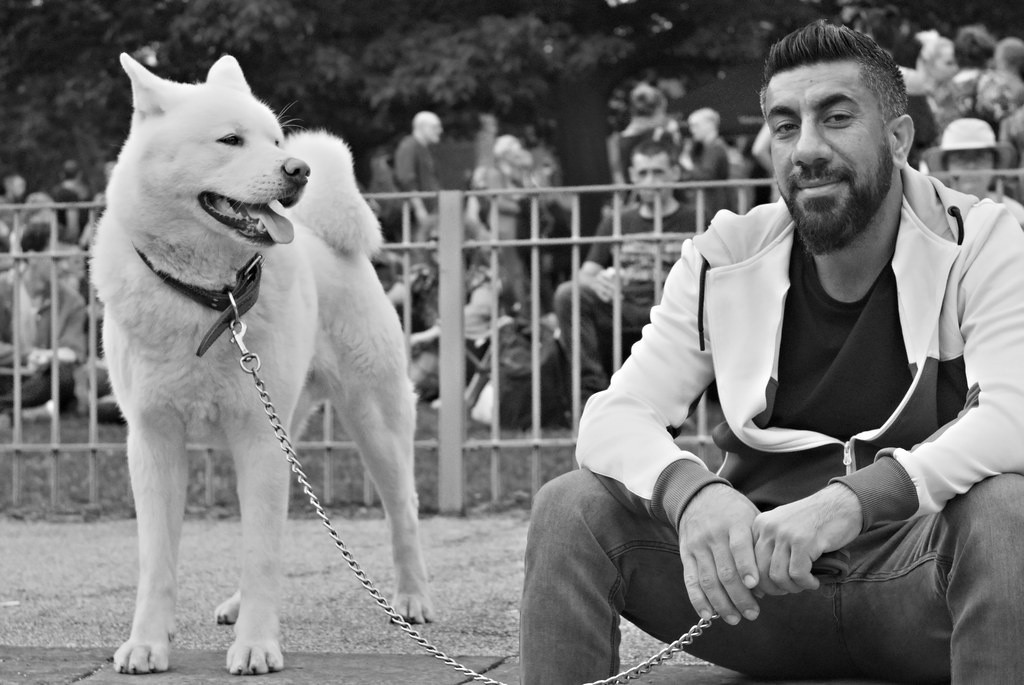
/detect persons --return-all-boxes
[378,18,1024,434]
[519,26,1024,685]
[0,155,130,430]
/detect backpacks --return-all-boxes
[466,319,571,430]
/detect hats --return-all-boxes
[940,118,997,151]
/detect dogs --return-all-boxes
[89,52,435,676]
